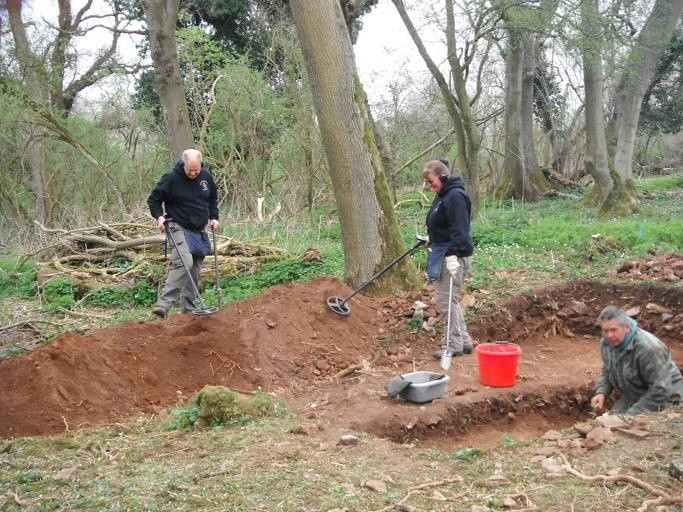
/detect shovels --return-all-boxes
[440,275,454,370]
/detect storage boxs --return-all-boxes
[398,371,450,404]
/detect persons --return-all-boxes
[419,158,473,360]
[589,305,682,415]
[146,149,219,318]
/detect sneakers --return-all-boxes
[463,344,473,354]
[152,307,167,318]
[433,350,463,359]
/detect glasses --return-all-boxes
[424,175,442,183]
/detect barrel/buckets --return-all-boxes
[475,341,522,387]
